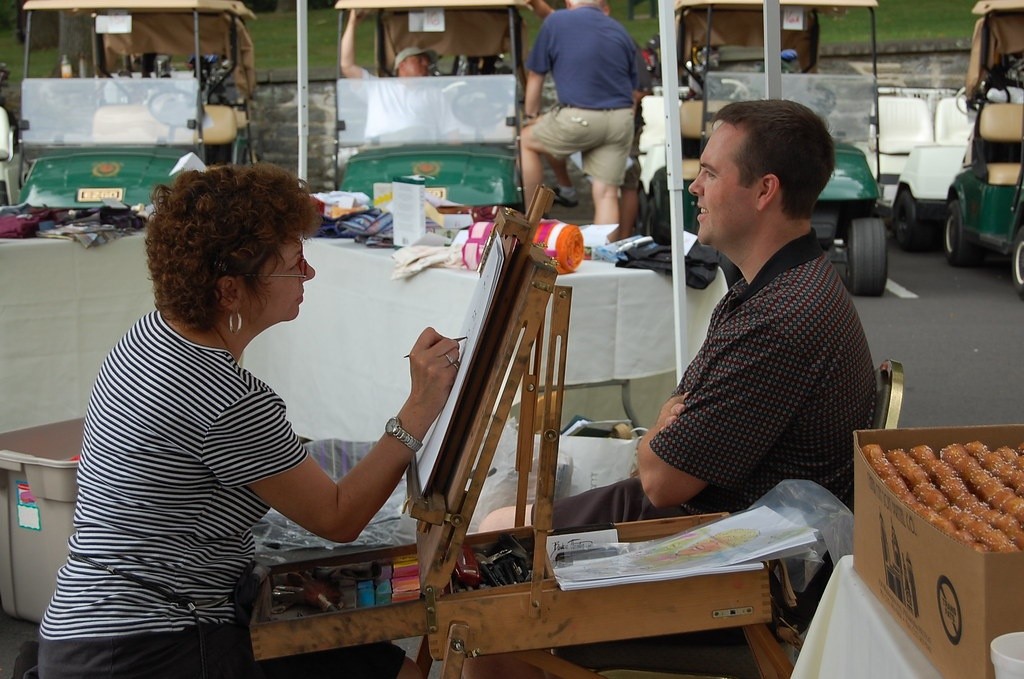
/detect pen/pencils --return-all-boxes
[403,337,467,358]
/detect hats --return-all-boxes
[393,46,439,71]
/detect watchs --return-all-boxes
[385,418,423,453]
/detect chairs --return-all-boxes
[935,97,972,147]
[552,358,903,679]
[872,95,933,155]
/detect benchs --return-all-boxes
[681,101,734,181]
[92,104,239,146]
[978,104,1024,184]
[441,89,516,143]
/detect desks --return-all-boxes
[781,552,942,679]
[237,234,729,439]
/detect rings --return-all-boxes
[452,364,459,370]
[445,353,453,365]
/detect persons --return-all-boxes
[341,9,472,147]
[460,98,877,679]
[37,164,461,679]
[520,0,650,243]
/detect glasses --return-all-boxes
[226,257,309,285]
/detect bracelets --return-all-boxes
[522,113,541,120]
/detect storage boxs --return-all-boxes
[0,417,86,623]
[392,174,472,244]
[853,421,1024,679]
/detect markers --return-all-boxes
[480,557,526,588]
[555,547,618,562]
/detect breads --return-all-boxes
[863,441,1024,552]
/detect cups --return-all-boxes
[989,632,1024,679]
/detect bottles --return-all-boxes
[605,422,632,440]
[60,54,72,78]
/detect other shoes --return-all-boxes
[551,186,579,208]
